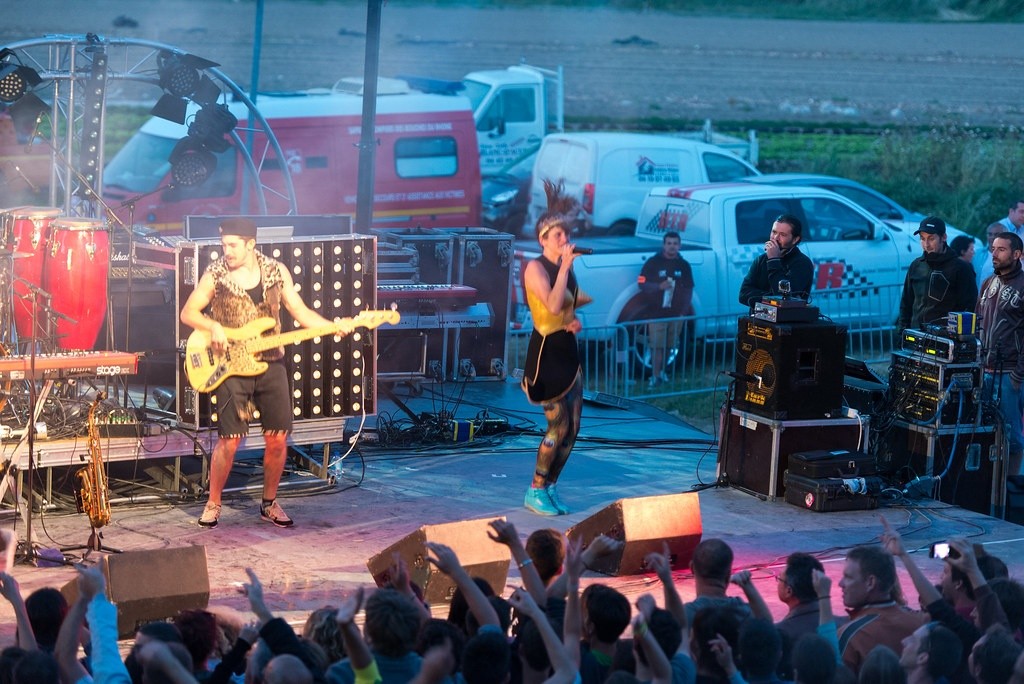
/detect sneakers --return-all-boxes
[525,485,559,516]
[260,500,293,528]
[545,484,569,515]
[198,501,221,528]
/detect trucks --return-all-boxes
[456,54,761,172]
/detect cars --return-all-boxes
[474,146,545,234]
[737,174,988,290]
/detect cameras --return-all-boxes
[929,543,959,559]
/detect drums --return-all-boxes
[2,205,113,352]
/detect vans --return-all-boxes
[86,76,482,230]
[519,133,764,238]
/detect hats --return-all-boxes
[914,216,946,235]
[535,211,578,238]
[219,221,257,236]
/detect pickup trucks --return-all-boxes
[514,179,924,383]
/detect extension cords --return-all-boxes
[841,406,859,419]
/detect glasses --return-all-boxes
[776,574,790,587]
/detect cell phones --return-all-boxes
[573,247,593,255]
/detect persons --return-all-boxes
[974,232,1024,479]
[738,214,815,312]
[974,222,1008,297]
[0,513,1023,684]
[998,200,1024,272]
[638,232,694,386]
[950,235,974,262]
[897,216,978,348]
[179,220,355,528]
[523,211,593,516]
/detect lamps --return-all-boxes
[153,49,237,190]
[0,47,51,143]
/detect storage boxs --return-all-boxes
[717,401,1010,516]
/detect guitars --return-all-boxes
[183,301,402,394]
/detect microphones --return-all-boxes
[10,161,41,194]
[722,371,757,382]
[25,111,43,154]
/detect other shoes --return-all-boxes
[660,370,669,384]
[649,376,656,386]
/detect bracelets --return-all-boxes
[411,593,417,599]
[582,561,589,569]
[519,560,531,569]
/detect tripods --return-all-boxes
[0,267,126,569]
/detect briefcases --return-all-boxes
[788,449,877,479]
[783,470,880,512]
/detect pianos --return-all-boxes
[0,348,140,569]
[376,284,479,426]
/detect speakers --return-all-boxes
[366,516,511,606]
[565,491,702,576]
[59,545,211,641]
[733,317,848,422]
